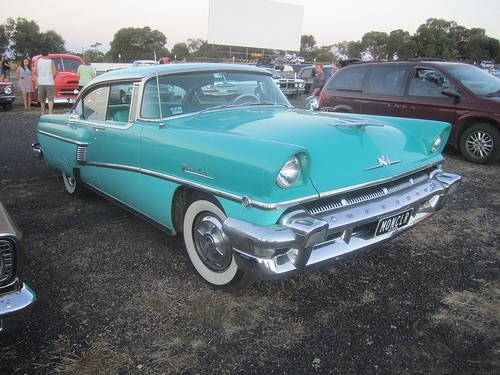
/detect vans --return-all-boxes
[318,61,500,164]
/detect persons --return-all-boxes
[16,58,35,110]
[284,60,341,112]
[75,56,97,91]
[35,49,57,116]
[0,54,10,83]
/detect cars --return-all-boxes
[29,54,97,105]
[479,61,500,80]
[0,203,40,333]
[31,62,462,292]
[0,65,16,111]
[249,55,335,99]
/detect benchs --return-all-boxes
[113,102,227,122]
